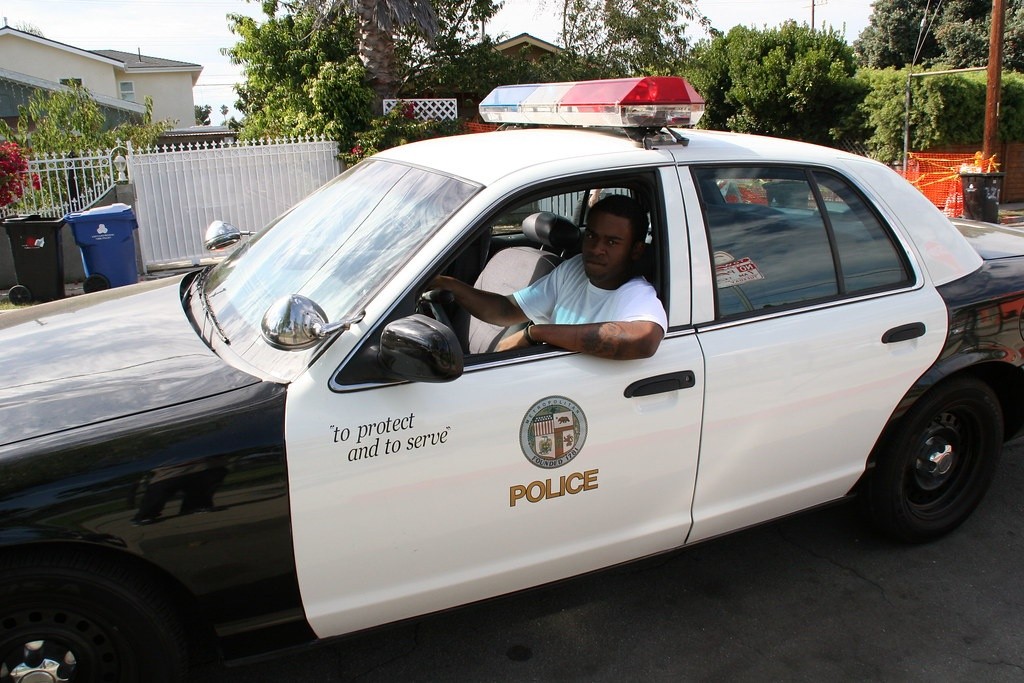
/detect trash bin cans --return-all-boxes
[64,202,139,294]
[762,181,809,208]
[959,172,1005,224]
[0,214,66,306]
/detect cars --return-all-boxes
[0,75,1024,683]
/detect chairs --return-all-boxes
[491,212,581,355]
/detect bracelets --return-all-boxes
[524,321,543,346]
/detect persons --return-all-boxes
[426,194,667,360]
